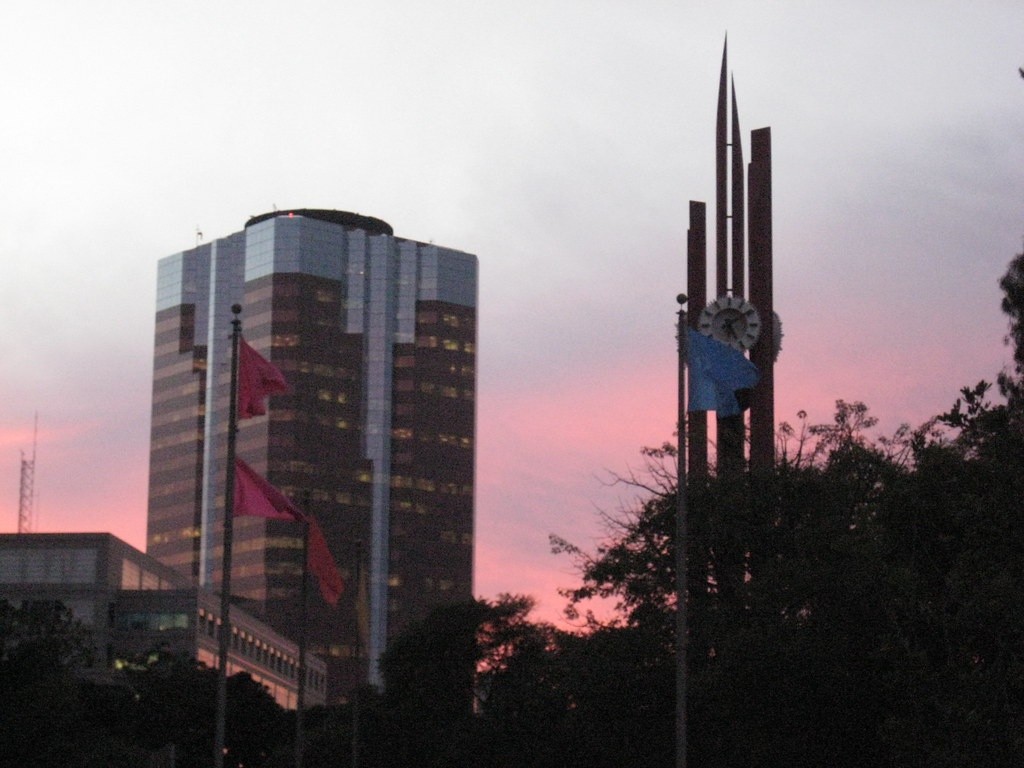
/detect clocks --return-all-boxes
[698,296,760,352]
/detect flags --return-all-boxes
[685,330,761,420]
[233,333,347,606]
